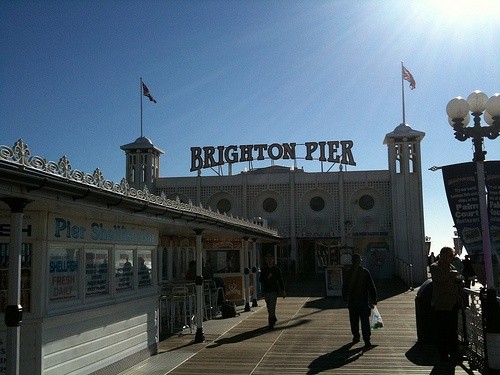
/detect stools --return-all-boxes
[160,279,224,335]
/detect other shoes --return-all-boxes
[274,317,277,326]
[267,326,273,330]
[443,357,451,363]
[365,342,372,349]
[452,353,463,360]
[352,337,360,342]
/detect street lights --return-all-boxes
[445,89,500,290]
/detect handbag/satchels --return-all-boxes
[222,307,236,319]
[371,304,384,329]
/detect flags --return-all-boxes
[403,66,415,90]
[142,81,157,103]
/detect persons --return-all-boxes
[101,258,108,273]
[123,257,132,286]
[342,253,377,348]
[259,253,286,330]
[428,252,436,273]
[185,257,213,321]
[430,247,466,375]
[137,257,150,286]
[451,255,474,308]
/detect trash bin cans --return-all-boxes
[484,302,500,375]
